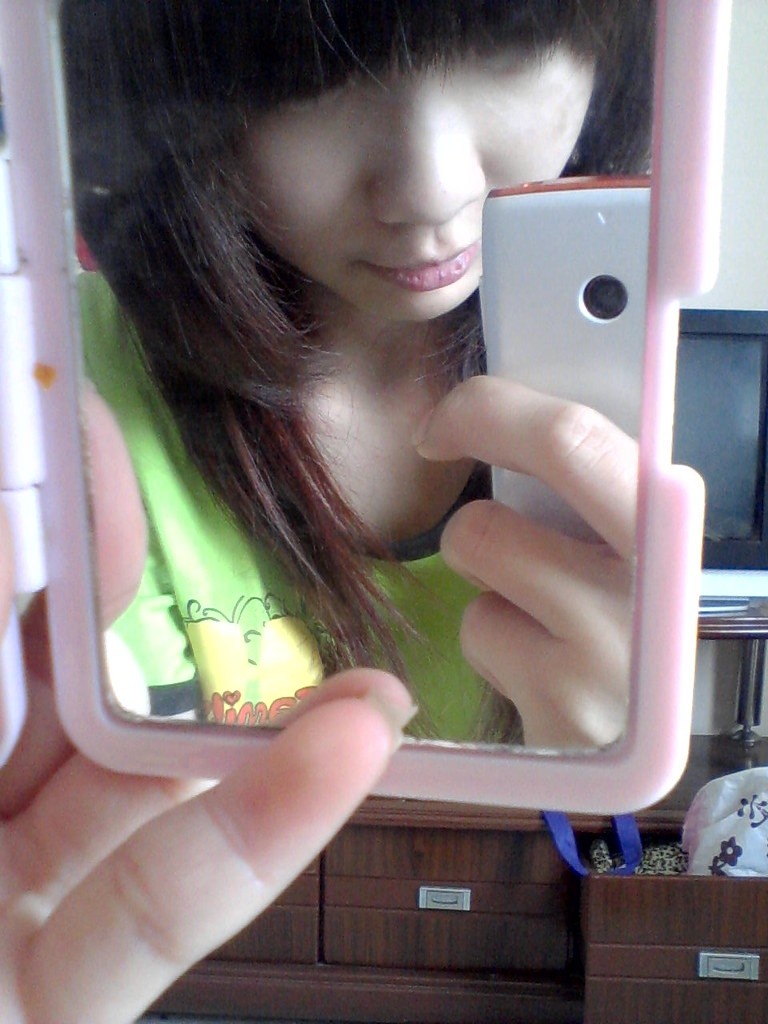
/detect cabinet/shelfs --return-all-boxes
[148,734,768,1024]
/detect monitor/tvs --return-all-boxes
[670,310,768,614]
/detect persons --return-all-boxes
[0,588,404,1024]
[72,0,658,756]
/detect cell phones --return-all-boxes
[480,172,652,544]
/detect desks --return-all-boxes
[698,596,768,746]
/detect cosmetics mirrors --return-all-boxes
[0,0,729,817]
